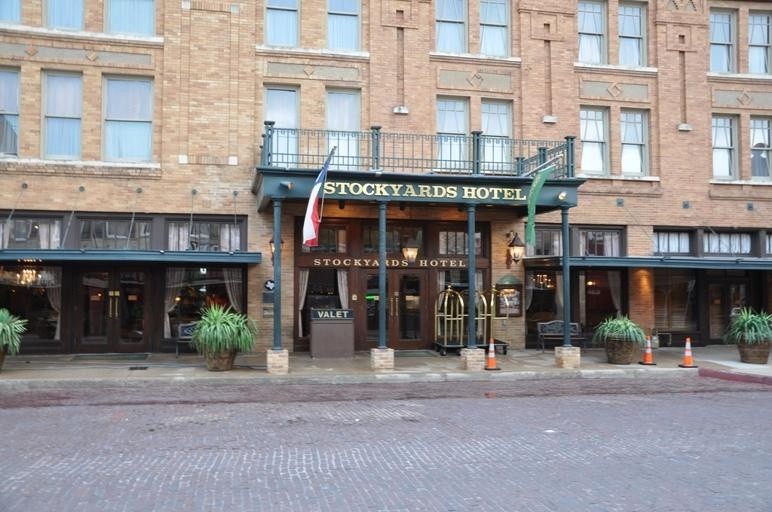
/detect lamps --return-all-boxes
[268,232,285,265]
[400,235,419,264]
[505,228,526,265]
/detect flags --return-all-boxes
[302,151,331,247]
[525,166,555,247]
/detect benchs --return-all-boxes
[535,319,585,354]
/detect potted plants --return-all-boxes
[191,301,260,373]
[2,306,29,370]
[721,305,772,364]
[593,311,648,366]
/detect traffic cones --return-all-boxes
[485,338,501,371]
[679,337,698,368]
[639,336,656,366]
